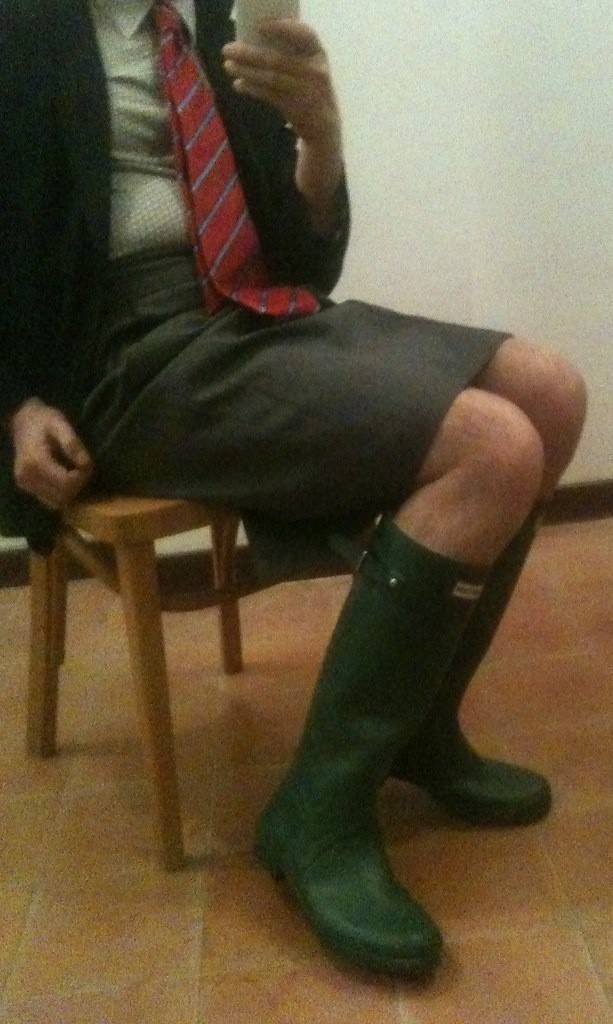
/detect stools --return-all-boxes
[24,495,287,870]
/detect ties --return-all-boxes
[149,0,320,317]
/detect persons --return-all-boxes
[0,0,590,980]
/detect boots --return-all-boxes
[379,499,554,825]
[251,511,443,976]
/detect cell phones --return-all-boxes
[233,0,300,48]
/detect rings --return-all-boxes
[270,72,281,92]
